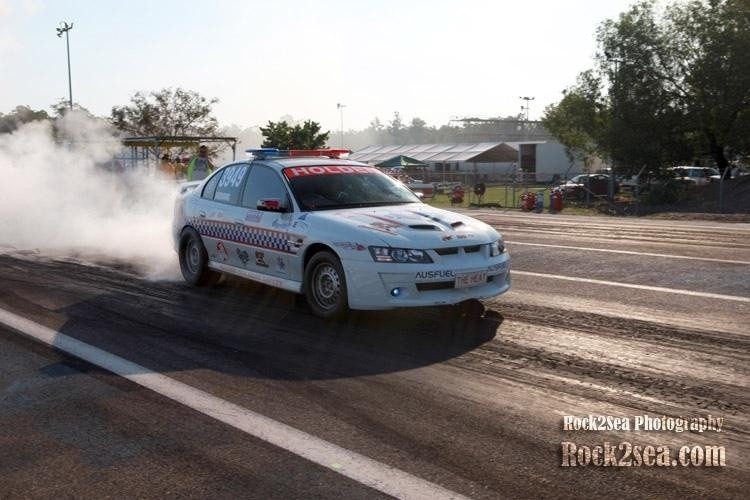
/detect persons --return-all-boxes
[157,145,218,181]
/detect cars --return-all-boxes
[435,180,463,193]
[172,148,511,319]
[393,175,423,184]
[554,173,619,200]
[620,164,750,196]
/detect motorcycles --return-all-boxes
[517,190,563,210]
